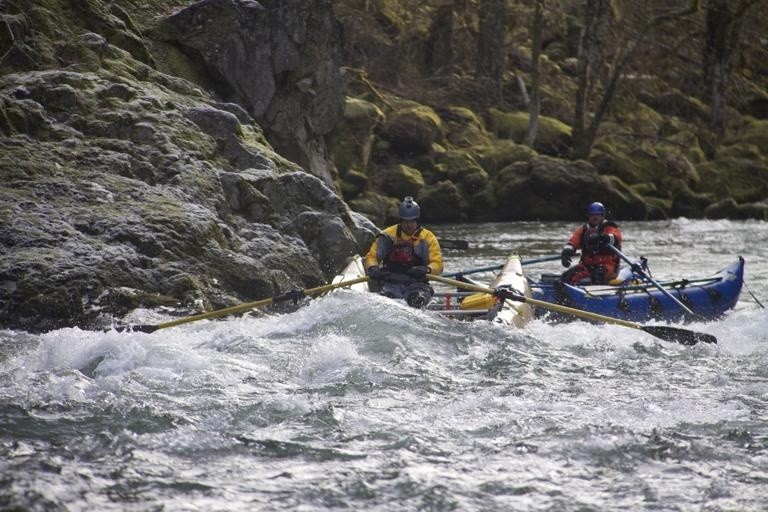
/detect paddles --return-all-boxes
[105,271,391,334]
[422,272,717,346]
[604,242,710,324]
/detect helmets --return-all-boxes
[587,202,605,214]
[398,196,420,224]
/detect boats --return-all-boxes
[331,250,533,332]
[451,252,745,324]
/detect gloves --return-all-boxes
[591,232,610,248]
[407,265,430,278]
[561,248,572,267]
[368,267,379,279]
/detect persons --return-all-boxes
[364,196,444,308]
[560,201,623,286]
